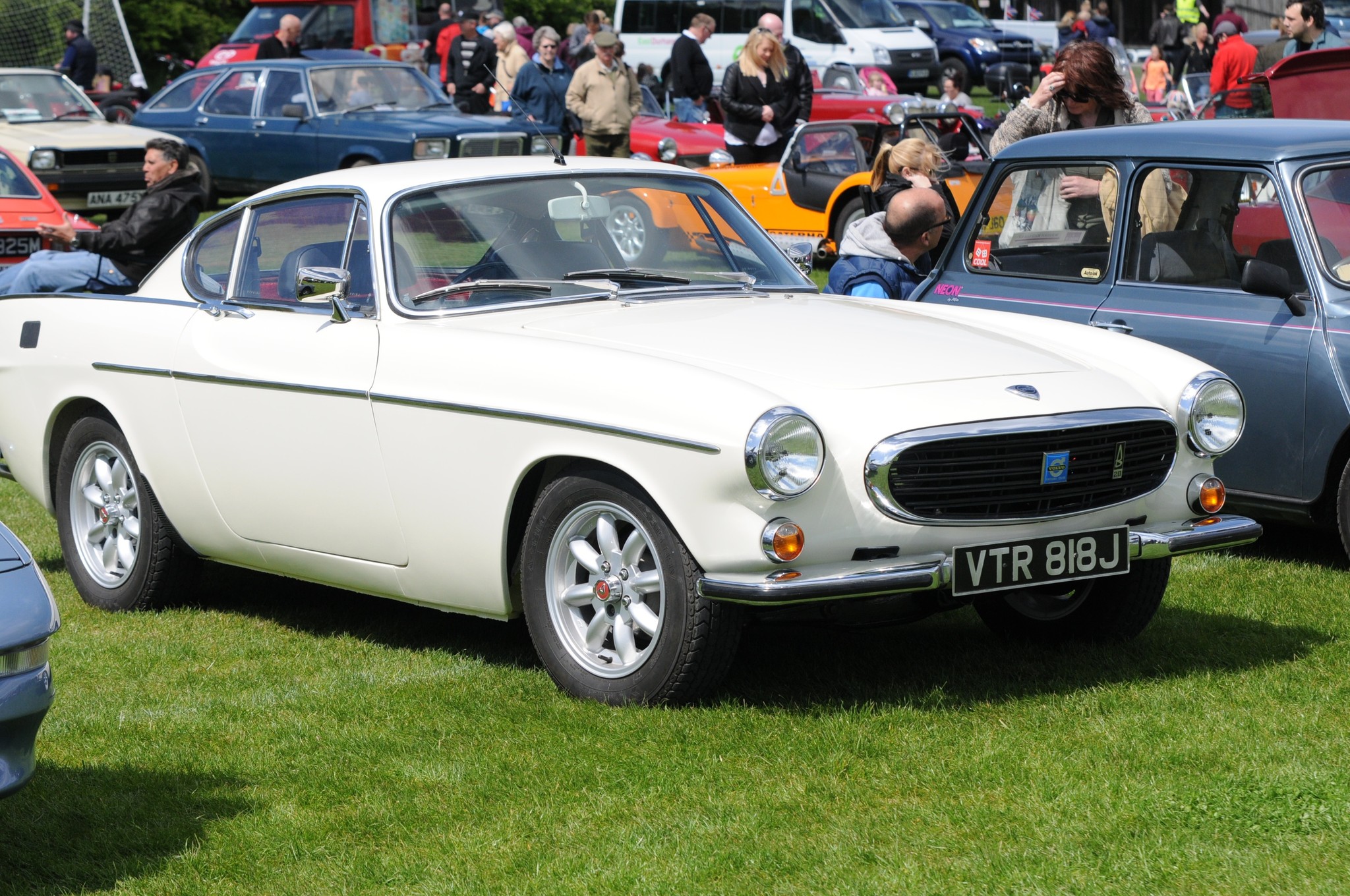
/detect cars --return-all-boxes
[908,119,1349,561]
[0,145,100,273]
[127,50,562,212]
[891,0,1041,98]
[803,61,982,154]
[0,67,213,232]
[1,158,1261,710]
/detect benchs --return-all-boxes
[992,251,1111,281]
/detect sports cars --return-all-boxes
[571,86,728,169]
[597,120,1014,268]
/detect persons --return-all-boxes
[253,13,304,99]
[0,138,208,297]
[564,30,643,159]
[509,26,585,155]
[823,1,1350,302]
[670,13,716,124]
[56,20,97,91]
[425,0,662,115]
[717,27,789,164]
[757,12,813,163]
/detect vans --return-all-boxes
[611,0,940,98]
[192,0,502,102]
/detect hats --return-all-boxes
[485,9,506,20]
[594,32,618,47]
[452,7,480,23]
[63,20,83,32]
[1213,20,1237,36]
[494,21,515,41]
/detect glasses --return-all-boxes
[703,25,714,35]
[898,166,933,177]
[1055,88,1092,103]
[539,45,558,48]
[747,28,771,42]
[925,216,953,234]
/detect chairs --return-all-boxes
[345,90,372,111]
[470,242,613,306]
[1140,230,1242,290]
[934,133,971,164]
[53,210,199,293]
[275,239,415,310]
[1254,235,1343,293]
[272,80,325,118]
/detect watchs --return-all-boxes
[70,231,80,247]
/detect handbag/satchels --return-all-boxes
[1067,196,1104,229]
[565,109,585,141]
